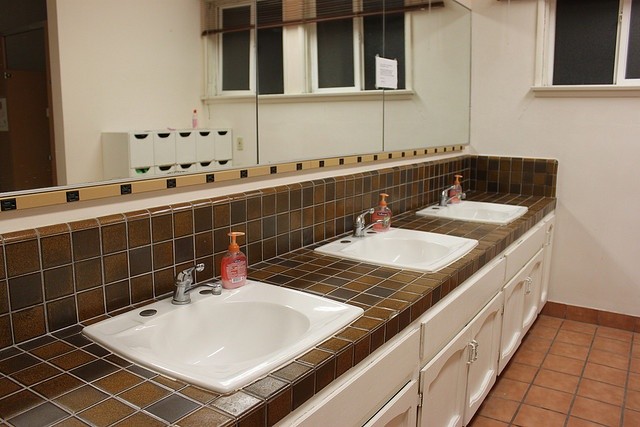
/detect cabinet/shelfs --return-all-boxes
[100,128,232,179]
[418,257,507,426]
[284,325,420,426]
[498,212,557,377]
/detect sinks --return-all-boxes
[83,279,364,392]
[415,200,528,225]
[313,226,479,273]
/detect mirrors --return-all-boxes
[0,1,471,201]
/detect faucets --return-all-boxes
[439,184,466,207]
[171,262,205,304]
[353,207,388,236]
